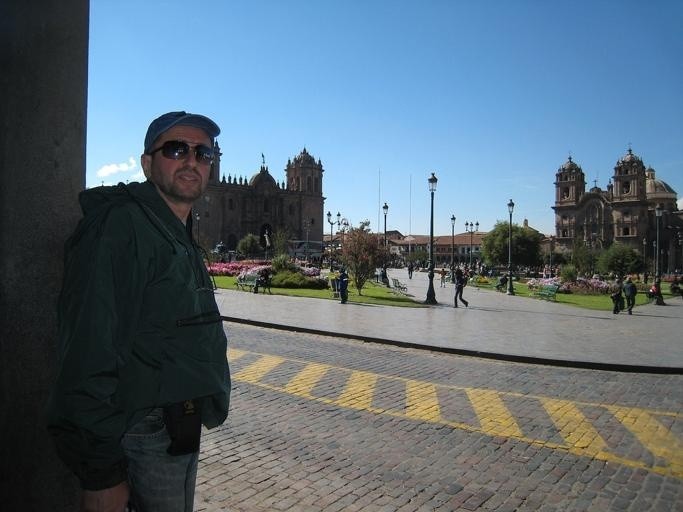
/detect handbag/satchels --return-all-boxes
[610,293,619,303]
[163,399,201,456]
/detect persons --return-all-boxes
[339,268,348,304]
[543,267,548,279]
[439,262,499,308]
[414,263,419,270]
[48,109,232,512]
[408,262,413,279]
[644,271,649,284]
[610,275,637,316]
[671,275,680,287]
[495,274,509,292]
[651,280,663,302]
[252,268,269,293]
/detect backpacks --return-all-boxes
[625,285,633,297]
[462,276,468,287]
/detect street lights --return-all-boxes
[303,215,315,257]
[382,202,389,270]
[639,203,672,306]
[326,210,342,271]
[196,216,201,244]
[466,220,479,266]
[423,173,439,305]
[506,198,516,295]
[449,214,456,267]
[549,234,554,280]
[264,229,270,260]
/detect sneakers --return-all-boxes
[453,301,468,308]
[338,299,348,304]
[613,311,633,315]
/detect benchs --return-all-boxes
[231,272,273,295]
[391,277,408,296]
[532,285,559,303]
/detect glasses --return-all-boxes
[147,140,215,166]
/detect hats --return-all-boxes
[144,111,221,148]
